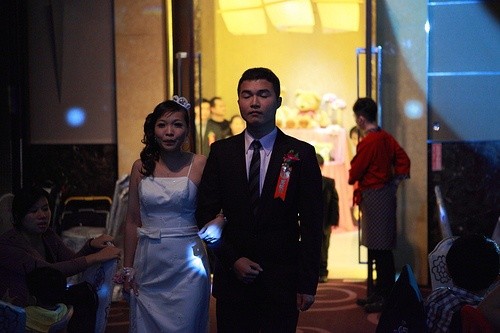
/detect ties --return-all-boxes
[248,139,264,200]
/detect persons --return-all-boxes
[230,116,245,137]
[0,187,119,332]
[349,125,363,146]
[204,96,231,155]
[348,97,411,312]
[120,95,224,333]
[313,153,339,283]
[198,68,325,333]
[425,234,500,333]
[194,98,215,155]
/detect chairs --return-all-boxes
[427,236,461,292]
[0,193,117,333]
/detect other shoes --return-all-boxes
[356,294,377,306]
[364,298,385,312]
[319,275,328,282]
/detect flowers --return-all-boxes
[279,149,301,173]
